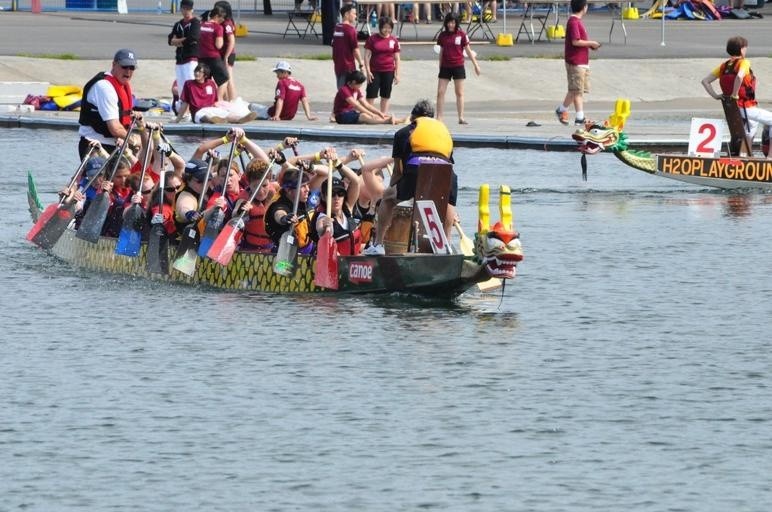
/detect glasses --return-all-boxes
[122,66,135,70]
[141,188,155,194]
[164,186,181,192]
[332,191,344,197]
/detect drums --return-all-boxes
[383,201,413,256]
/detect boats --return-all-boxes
[571,97,772,195]
[24,169,525,300]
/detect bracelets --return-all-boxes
[358,64,364,68]
[730,94,739,100]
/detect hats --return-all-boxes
[272,62,291,72]
[86,158,105,176]
[182,0,193,8]
[183,160,213,181]
[114,49,137,67]
[322,177,346,191]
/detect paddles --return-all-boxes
[114,124,158,256]
[26,144,100,240]
[456,223,502,291]
[273,160,309,278]
[314,159,338,290]
[31,141,121,249]
[145,145,170,275]
[197,131,238,256]
[172,152,215,277]
[75,116,142,243]
[207,152,276,266]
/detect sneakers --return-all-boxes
[459,121,468,124]
[238,112,256,123]
[555,106,569,125]
[363,243,385,255]
[575,116,594,125]
[211,116,226,123]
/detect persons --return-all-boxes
[435,13,481,124]
[213,0,238,102]
[172,78,185,114]
[193,6,232,108]
[53,109,461,257]
[330,3,366,122]
[294,0,317,12]
[237,61,317,121]
[341,0,499,27]
[333,70,413,124]
[364,16,402,116]
[554,0,602,126]
[359,98,459,256]
[77,49,142,168]
[700,36,772,160]
[166,0,198,110]
[168,62,258,124]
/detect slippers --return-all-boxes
[526,121,541,127]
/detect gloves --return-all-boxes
[150,213,163,224]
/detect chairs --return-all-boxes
[515,0,553,42]
[283,0,319,40]
[464,0,495,41]
